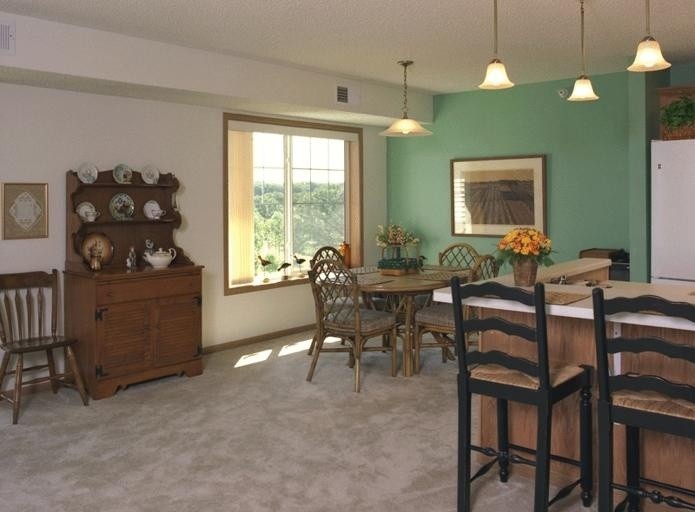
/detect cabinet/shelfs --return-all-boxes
[62,169,205,401]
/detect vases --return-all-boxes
[512,260,538,287]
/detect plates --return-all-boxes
[76,163,161,185]
[75,202,95,222]
[108,193,134,219]
[143,198,161,219]
[80,232,113,267]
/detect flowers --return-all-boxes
[492,226,559,268]
[375,218,421,266]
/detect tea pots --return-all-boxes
[144,248,177,268]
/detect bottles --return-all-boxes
[339,243,351,269]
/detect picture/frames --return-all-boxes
[2,183,48,239]
[450,154,546,239]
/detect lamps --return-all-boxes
[377,60,433,138]
[477,1,514,90]
[625,0,672,72]
[565,0,599,102]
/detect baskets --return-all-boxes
[378,244,423,276]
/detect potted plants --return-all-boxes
[654,95,695,140]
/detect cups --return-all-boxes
[151,209,166,218]
[85,210,99,222]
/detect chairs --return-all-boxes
[0,269,89,425]
[305,240,500,392]
[450,275,595,512]
[591,287,694,512]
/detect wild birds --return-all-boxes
[258,256,272,267]
[277,263,291,272]
[294,256,306,264]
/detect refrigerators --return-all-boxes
[648,138,694,287]
